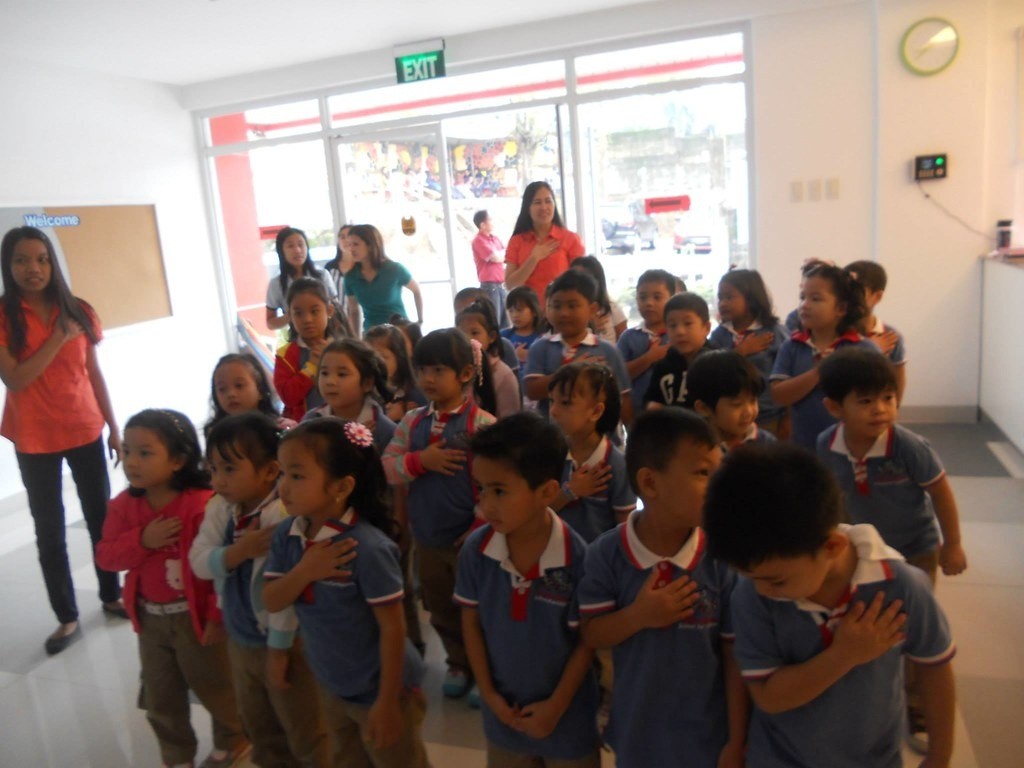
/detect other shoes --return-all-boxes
[199,739,253,768]
[907,706,930,754]
[102,604,130,620]
[44,621,81,654]
[442,659,475,697]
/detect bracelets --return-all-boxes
[562,480,579,502]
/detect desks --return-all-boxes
[977,249,1024,455]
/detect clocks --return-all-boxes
[900,17,960,78]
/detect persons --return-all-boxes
[0,227,133,654]
[93,179,969,768]
